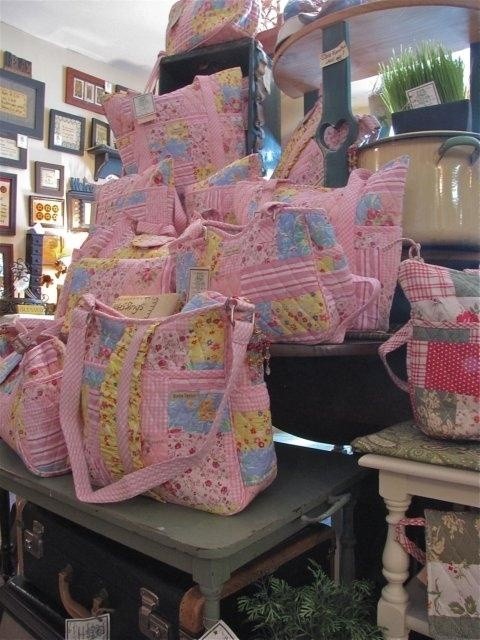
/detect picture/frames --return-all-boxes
[0,65,130,303]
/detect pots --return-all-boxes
[354,127,480,251]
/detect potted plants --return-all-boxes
[375,37,474,132]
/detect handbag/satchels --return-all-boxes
[398,243,480,323]
[165,0,263,57]
[395,508,480,640]
[0,314,71,476]
[59,290,278,516]
[379,319,480,443]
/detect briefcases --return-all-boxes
[10,496,336,640]
